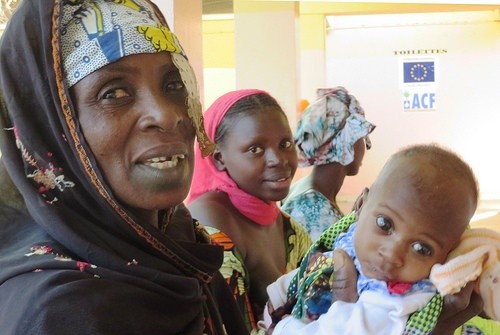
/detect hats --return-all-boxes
[59,0,189,89]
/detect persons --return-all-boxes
[259,144,480,334]
[184,88,314,327]
[278,86,376,243]
[0,0,489,335]
